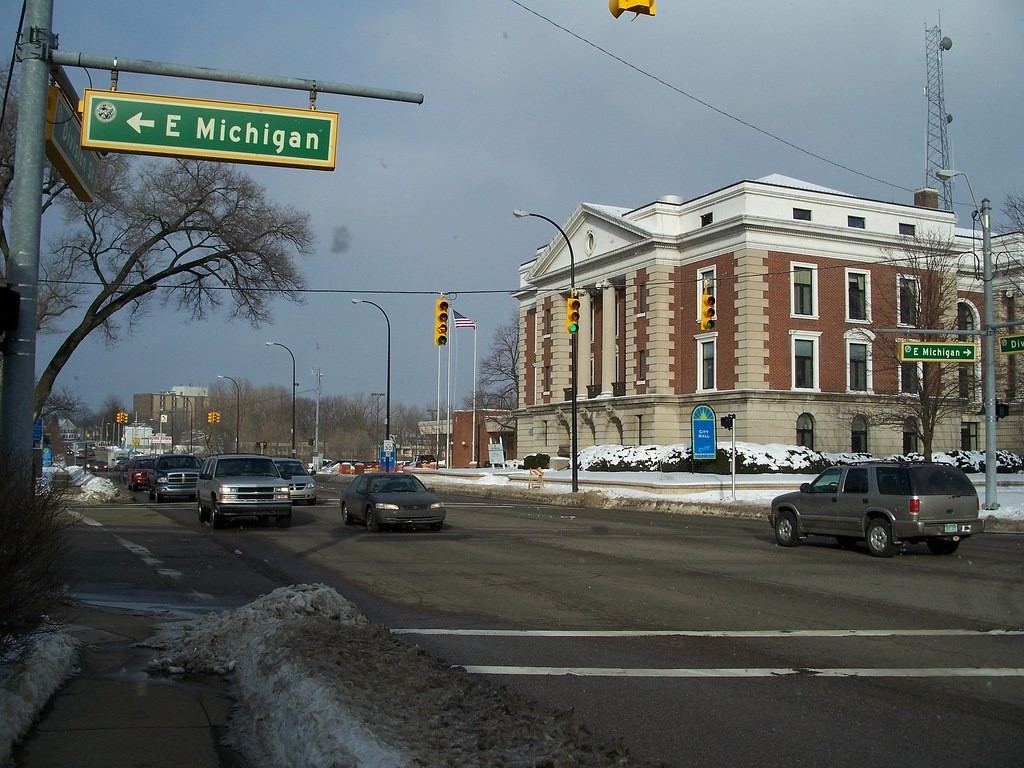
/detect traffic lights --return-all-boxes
[566,297,581,336]
[208,412,221,423]
[122,413,128,422]
[117,413,122,423]
[700,294,715,330]
[433,300,449,347]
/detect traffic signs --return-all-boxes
[79,91,337,170]
[900,334,1024,362]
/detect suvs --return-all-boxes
[147,455,205,502]
[768,459,986,556]
[195,453,293,528]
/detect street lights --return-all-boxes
[97,409,174,454]
[165,393,192,454]
[514,210,578,493]
[217,376,238,454]
[937,169,998,510]
[266,298,391,473]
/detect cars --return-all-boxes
[307,454,434,468]
[67,445,155,491]
[270,457,446,531]
